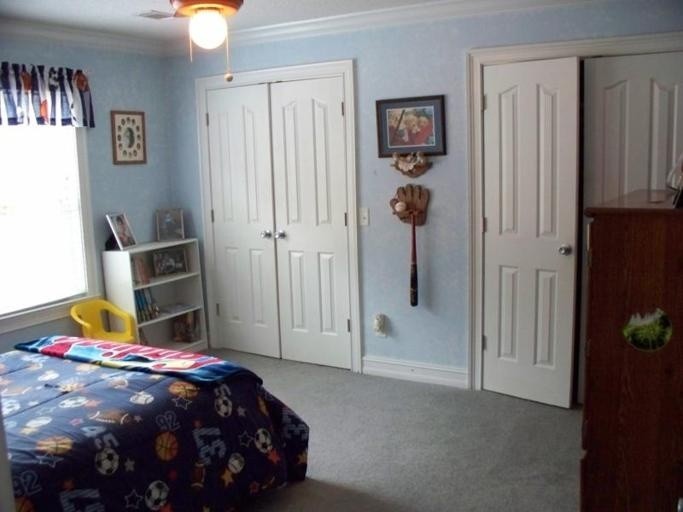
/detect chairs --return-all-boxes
[70,299,137,344]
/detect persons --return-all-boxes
[115,215,134,247]
[161,211,176,239]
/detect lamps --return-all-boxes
[170,0,244,83]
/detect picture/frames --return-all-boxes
[152,247,188,277]
[106,213,138,251]
[111,110,146,165]
[375,94,446,158]
[155,207,187,241]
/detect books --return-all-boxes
[131,254,202,346]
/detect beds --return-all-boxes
[0,334,309,512]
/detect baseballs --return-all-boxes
[394,202,406,212]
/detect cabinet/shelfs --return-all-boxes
[577,190,683,512]
[102,238,208,352]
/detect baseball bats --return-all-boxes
[409,208,417,305]
